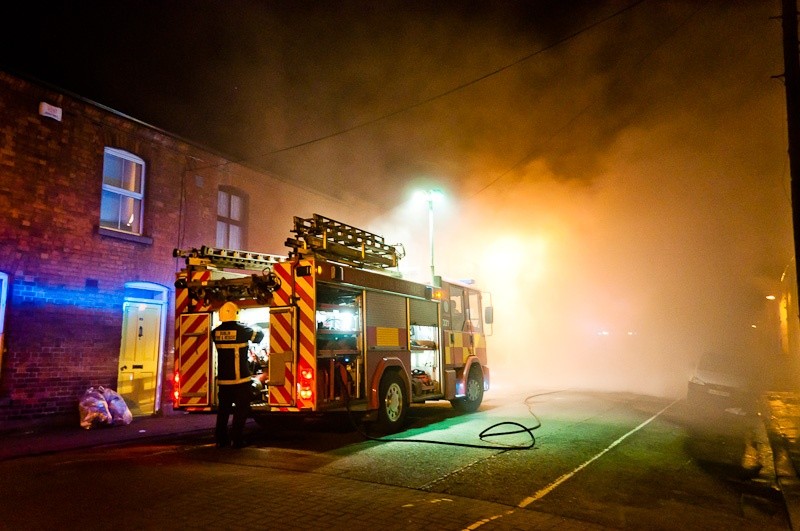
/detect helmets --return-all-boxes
[219,301,239,322]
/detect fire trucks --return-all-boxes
[171,212,493,443]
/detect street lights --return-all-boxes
[422,175,442,288]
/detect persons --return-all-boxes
[211,301,263,450]
